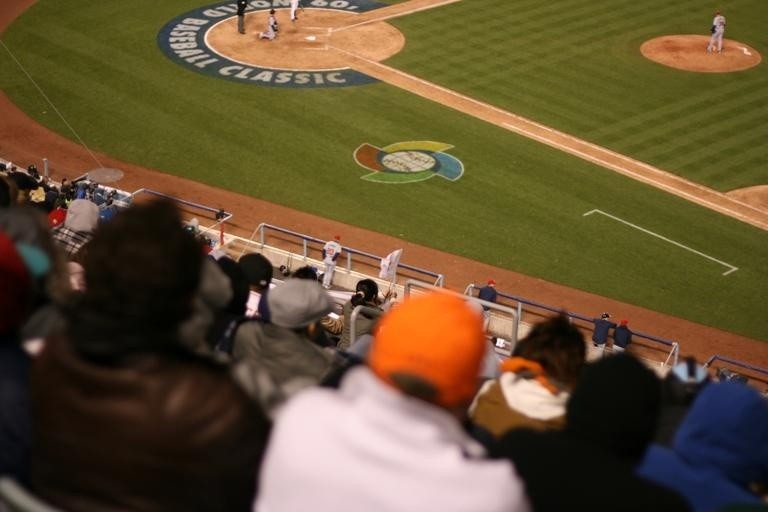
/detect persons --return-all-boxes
[0,161,767,510]
[235,0,249,36]
[289,1,301,23]
[258,8,280,41]
[708,10,727,54]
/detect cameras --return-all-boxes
[19,240,55,278]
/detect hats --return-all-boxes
[1,171,115,276]
[487,278,496,286]
[372,295,485,401]
[601,312,629,327]
[333,235,341,242]
[240,252,270,285]
[265,278,335,326]
